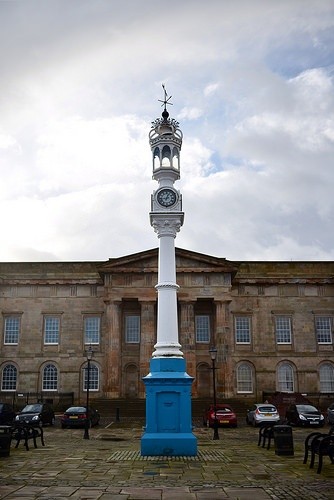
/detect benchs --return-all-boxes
[303,427,334,474]
[12,420,44,450]
[257,420,290,450]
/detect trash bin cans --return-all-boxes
[0,425,13,457]
[273,425,294,456]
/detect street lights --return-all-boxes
[84,350,93,441]
[208,345,220,440]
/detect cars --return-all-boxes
[16,403,55,428]
[203,403,237,428]
[246,403,280,427]
[61,406,101,429]
[284,404,326,428]
[0,403,14,425]
[326,407,334,425]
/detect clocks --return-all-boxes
[158,189,176,207]
